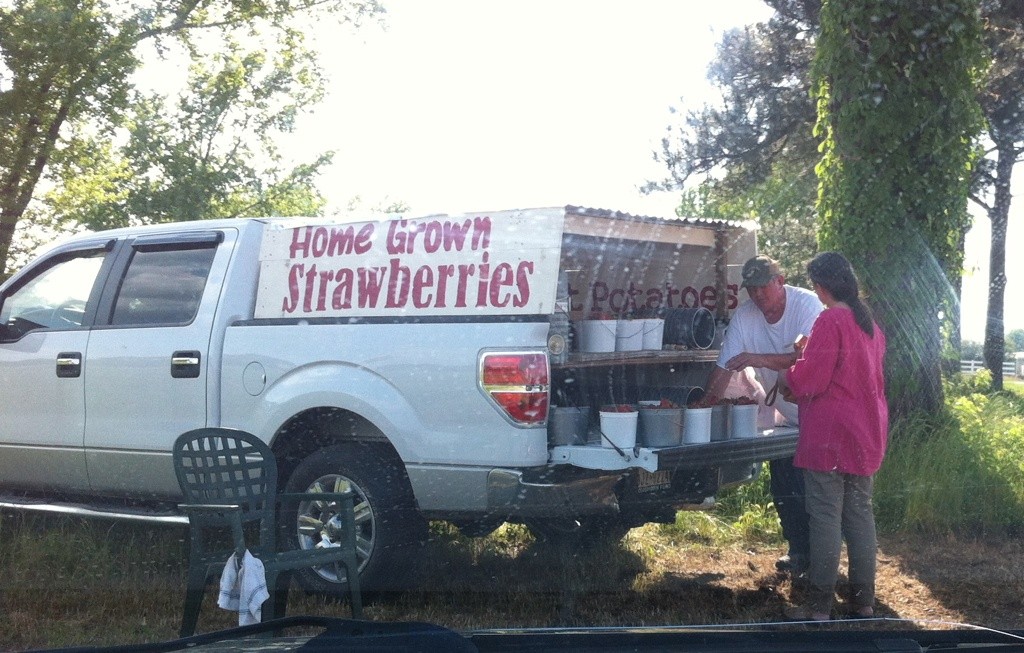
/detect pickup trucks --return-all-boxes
[0,218,802,604]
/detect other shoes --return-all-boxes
[837,599,874,617]
[775,553,809,570]
[784,604,829,620]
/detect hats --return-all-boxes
[739,255,780,289]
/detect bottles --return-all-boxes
[713,320,728,349]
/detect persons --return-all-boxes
[784,252,888,621]
[696,254,826,569]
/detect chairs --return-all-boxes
[172,428,362,640]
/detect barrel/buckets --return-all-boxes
[638,400,683,447]
[726,404,758,438]
[653,307,715,349]
[599,404,639,448]
[682,407,712,444]
[548,406,591,449]
[710,405,727,440]
[616,319,643,351]
[643,319,665,350]
[573,319,616,353]
